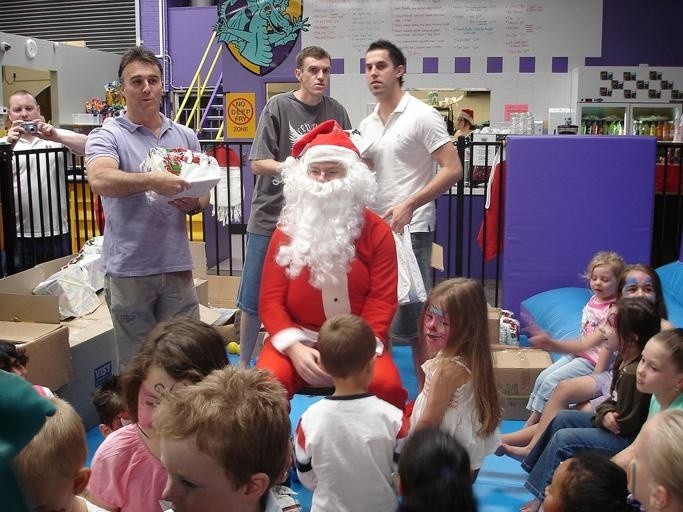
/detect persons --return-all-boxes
[84,46,213,383]
[1,249,682,511]
[234,46,354,369]
[247,119,410,410]
[1,91,88,277]
[350,38,463,346]
[454,107,476,138]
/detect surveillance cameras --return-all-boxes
[1,41,12,52]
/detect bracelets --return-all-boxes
[186,203,201,216]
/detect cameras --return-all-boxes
[21,122,38,134]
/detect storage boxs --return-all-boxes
[486,302,554,421]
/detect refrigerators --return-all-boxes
[569,64,682,193]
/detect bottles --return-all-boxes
[512,110,534,135]
[632,118,677,140]
[580,118,624,135]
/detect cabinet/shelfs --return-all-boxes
[570,65,683,193]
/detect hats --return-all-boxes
[286,119,360,165]
